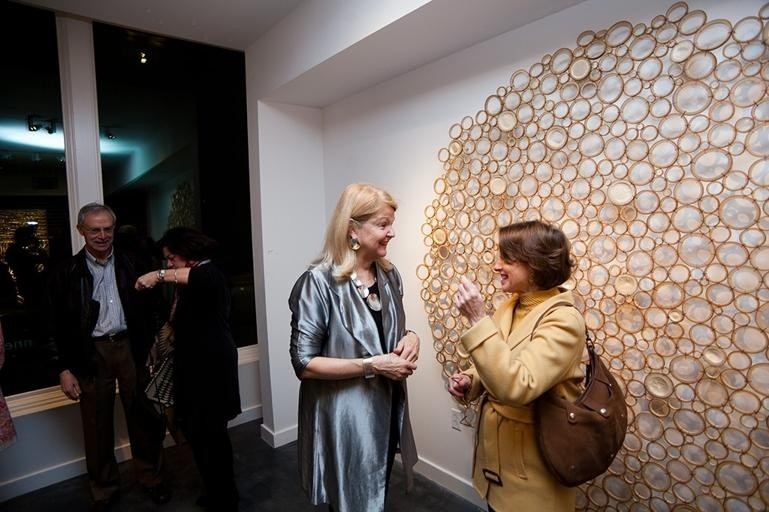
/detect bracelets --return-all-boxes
[362,357,375,379]
[174,269,177,282]
[157,269,166,284]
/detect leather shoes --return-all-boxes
[92,496,118,512]
[140,481,170,504]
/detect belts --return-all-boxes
[92,330,127,341]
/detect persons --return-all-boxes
[135,226,242,511]
[57,203,171,509]
[449,220,627,512]
[289,184,420,512]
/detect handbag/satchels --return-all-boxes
[530,301,627,487]
